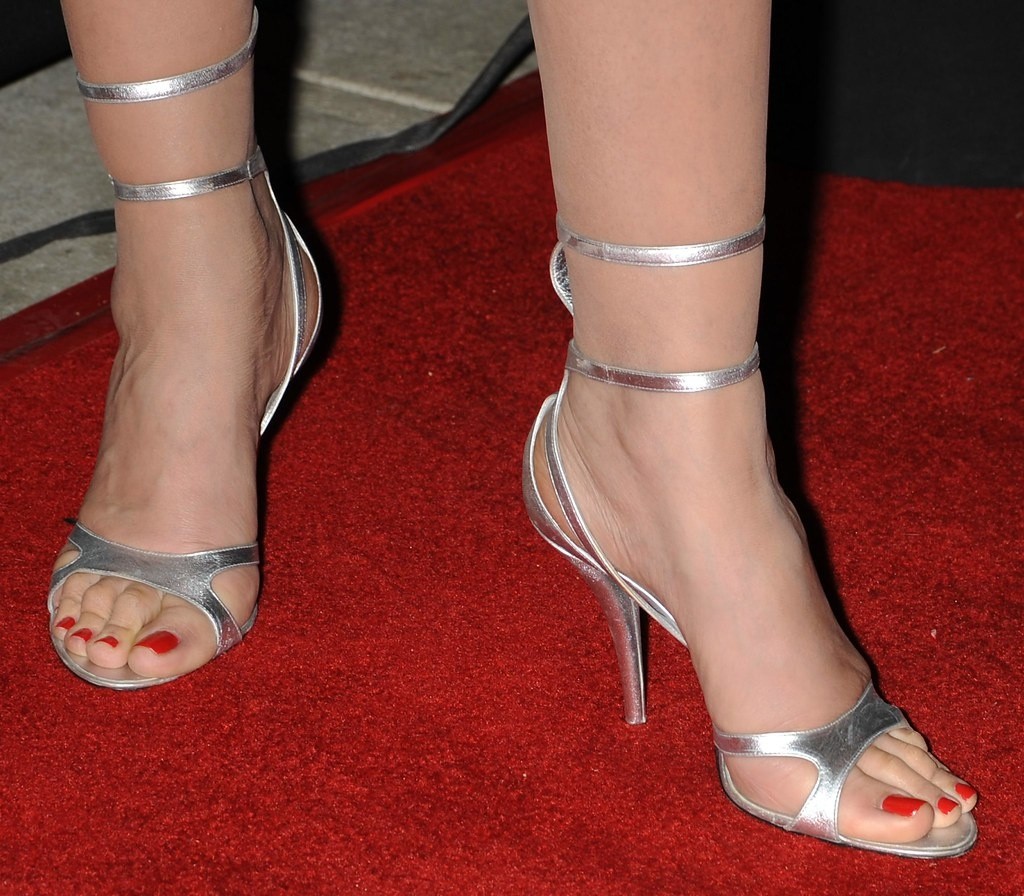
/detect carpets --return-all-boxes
[0,70,1024,896]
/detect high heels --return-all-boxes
[47,6,324,690]
[522,211,978,859]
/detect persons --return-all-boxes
[43,0,978,859]
